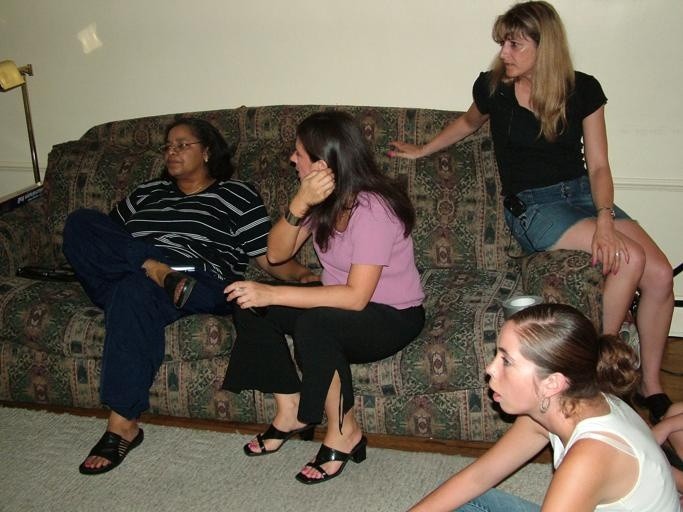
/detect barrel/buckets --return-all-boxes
[163,272,198,309]
[78,427,144,475]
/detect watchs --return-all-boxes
[596,207,616,219]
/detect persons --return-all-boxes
[652,401,682,494]
[218,110,426,485]
[387,0,675,425]
[407,303,680,510]
[62,117,320,476]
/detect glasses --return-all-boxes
[1,60,43,186]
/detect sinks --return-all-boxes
[1,105,618,462]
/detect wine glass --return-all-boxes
[615,250,620,256]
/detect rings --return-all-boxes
[615,250,620,256]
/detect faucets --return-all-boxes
[632,391,674,427]
[244,423,317,456]
[295,436,367,485]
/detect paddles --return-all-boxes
[1,403,552,511]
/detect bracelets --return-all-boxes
[284,209,305,227]
[284,210,302,226]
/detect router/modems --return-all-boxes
[503,295,544,320]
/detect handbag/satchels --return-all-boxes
[284,210,302,226]
[284,209,305,227]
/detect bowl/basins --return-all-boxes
[186,185,203,195]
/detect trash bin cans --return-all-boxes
[596,207,616,219]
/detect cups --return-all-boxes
[158,141,201,153]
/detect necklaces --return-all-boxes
[186,185,203,195]
